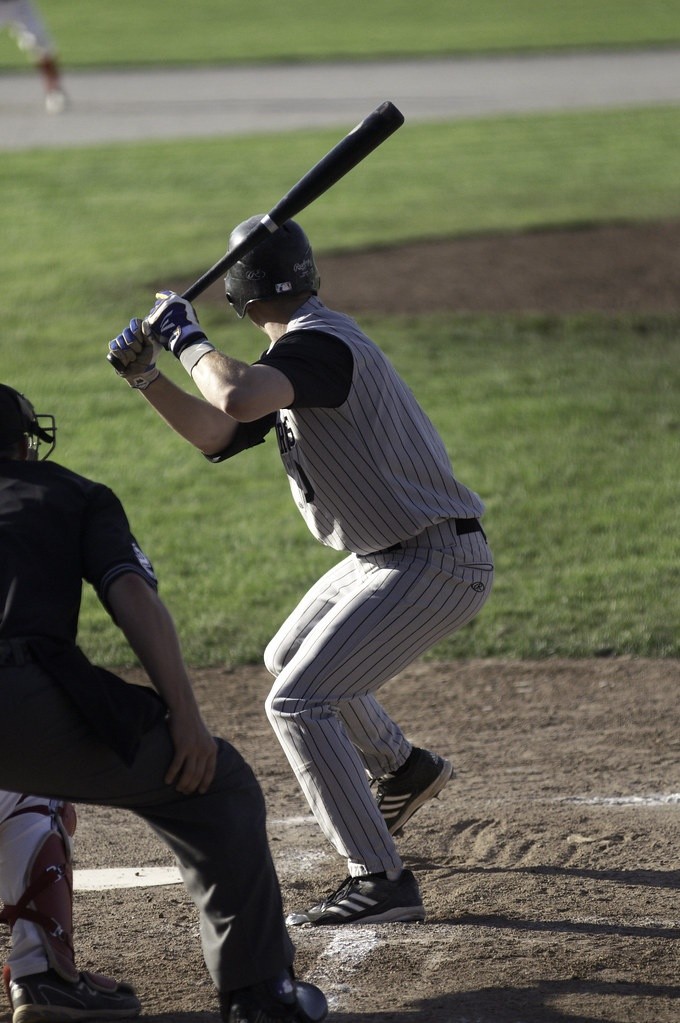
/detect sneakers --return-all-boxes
[366,746,457,836]
[288,867,425,926]
[10,973,142,1023]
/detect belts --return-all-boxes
[372,518,482,551]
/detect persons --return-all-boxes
[109,215,495,924]
[0,384,330,1023]
[0,0,68,112]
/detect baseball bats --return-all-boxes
[177,102,404,302]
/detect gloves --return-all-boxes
[140,290,215,379]
[107,317,164,390]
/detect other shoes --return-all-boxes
[44,89,68,116]
[217,969,329,1023]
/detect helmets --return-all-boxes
[222,213,321,319]
[0,383,56,461]
